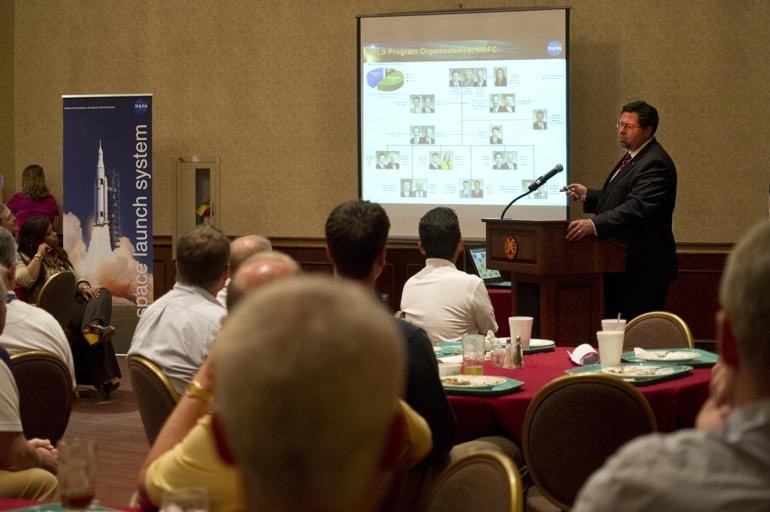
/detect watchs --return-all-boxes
[183,380,215,406]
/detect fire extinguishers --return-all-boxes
[198,199,210,224]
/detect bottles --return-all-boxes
[515,336,523,368]
[503,338,515,370]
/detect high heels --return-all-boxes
[79,320,117,347]
[99,376,120,402]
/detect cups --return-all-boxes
[508,316,534,351]
[460,333,486,376]
[602,318,627,331]
[596,330,626,368]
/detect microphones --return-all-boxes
[527,163,563,191]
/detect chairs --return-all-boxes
[126,351,179,450]
[522,369,660,512]
[624,310,695,348]
[422,450,522,512]
[35,270,78,327]
[393,309,405,320]
[8,348,76,450]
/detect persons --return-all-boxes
[431,153,440,168]
[469,182,482,197]
[560,101,678,324]
[535,112,546,130]
[472,69,485,85]
[490,95,503,111]
[1,204,52,289]
[127,228,228,396]
[134,251,431,512]
[450,71,461,86]
[462,69,476,87]
[408,95,422,113]
[400,181,413,197]
[490,127,502,144]
[228,236,273,270]
[496,69,505,86]
[12,217,122,403]
[208,273,407,512]
[494,153,505,169]
[410,127,424,143]
[441,153,451,168]
[500,96,513,111]
[386,154,398,167]
[323,202,533,510]
[1,164,60,237]
[421,127,433,144]
[525,181,546,199]
[0,273,60,504]
[400,208,500,351]
[572,220,767,511]
[377,153,386,168]
[409,180,425,197]
[423,96,433,113]
[502,154,516,169]
[460,182,471,197]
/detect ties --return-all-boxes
[617,153,632,175]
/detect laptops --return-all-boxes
[465,245,512,288]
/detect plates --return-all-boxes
[441,374,508,390]
[620,347,721,366]
[441,376,525,394]
[431,340,464,358]
[599,366,661,377]
[494,337,555,348]
[563,362,694,385]
[634,351,702,362]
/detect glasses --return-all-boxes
[616,121,643,131]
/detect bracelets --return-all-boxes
[79,285,91,292]
[31,253,43,262]
